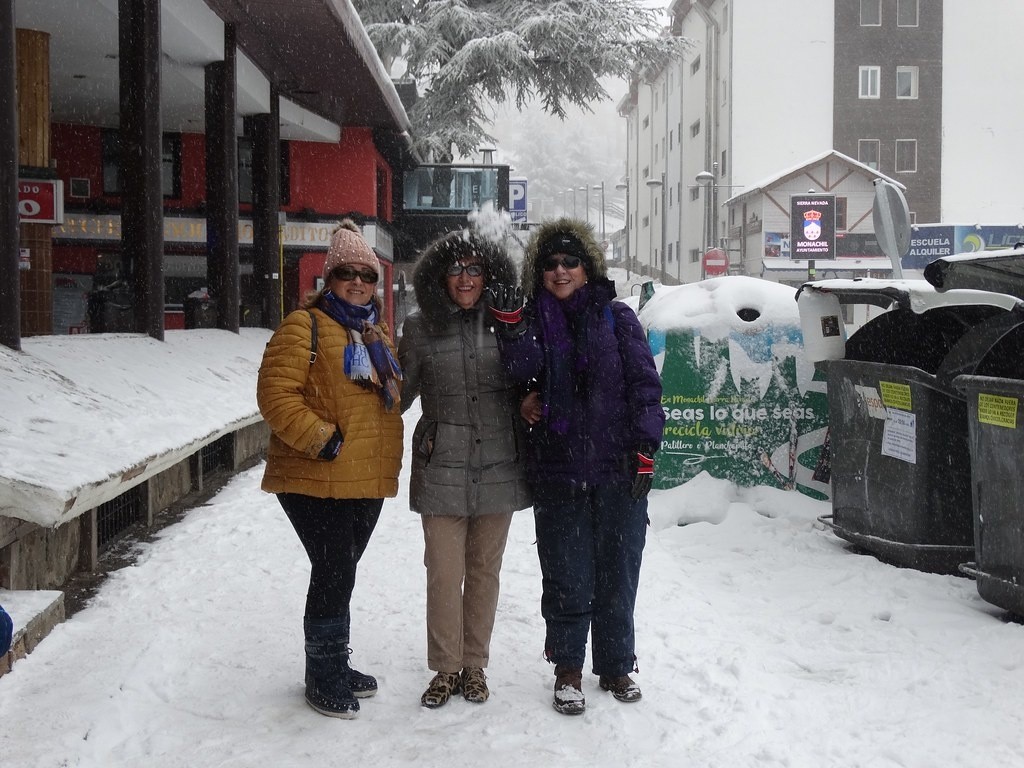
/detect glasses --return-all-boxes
[537,256,582,272]
[444,262,485,277]
[328,265,379,284]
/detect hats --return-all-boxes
[536,232,592,267]
[322,218,381,286]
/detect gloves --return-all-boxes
[485,284,527,338]
[318,427,343,462]
[628,441,655,501]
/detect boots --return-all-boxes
[304,616,379,720]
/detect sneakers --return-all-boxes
[599,675,643,703]
[420,666,490,709]
[552,665,586,715]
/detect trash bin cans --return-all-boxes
[795,249,1024,616]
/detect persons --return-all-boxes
[390,216,664,715]
[253,217,404,720]
